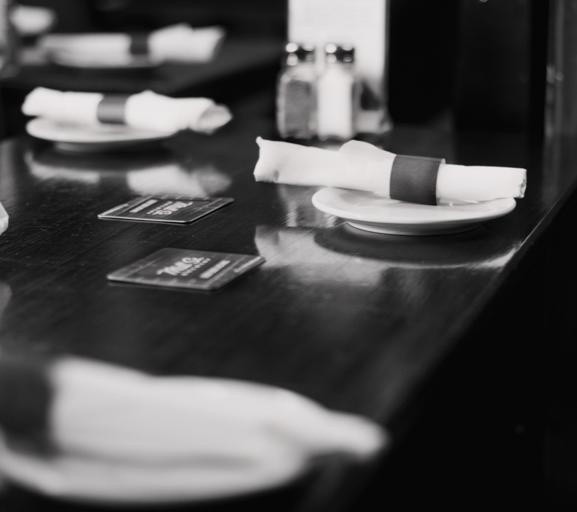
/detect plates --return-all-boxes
[47,48,164,72]
[2,447,310,507]
[311,186,516,240]
[25,119,184,146]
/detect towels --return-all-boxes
[254,132,528,203]
[19,83,234,136]
[33,26,225,69]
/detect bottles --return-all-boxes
[317,45,362,140]
[276,45,317,141]
